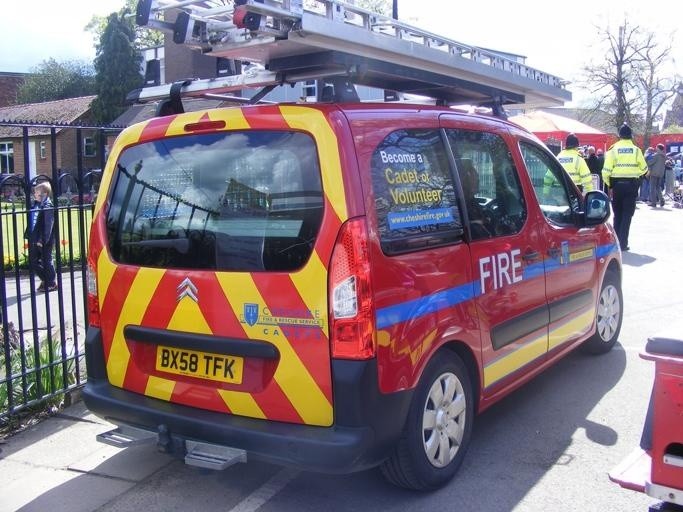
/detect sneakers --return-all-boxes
[37,281,58,292]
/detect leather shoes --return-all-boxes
[625,246,630,250]
[648,199,665,207]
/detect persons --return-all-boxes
[24,182,58,292]
[543,121,675,251]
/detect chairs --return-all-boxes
[455,155,481,200]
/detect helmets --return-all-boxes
[619,122,633,137]
[566,132,579,148]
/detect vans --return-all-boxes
[81,0,623,490]
